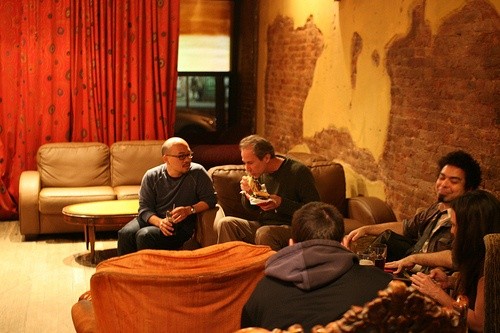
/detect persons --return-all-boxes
[118,137,218,256]
[343,150,481,275]
[240,201,389,333]
[217,135,320,252]
[385,189,500,333]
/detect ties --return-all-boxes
[428,209,448,238]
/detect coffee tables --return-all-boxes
[62,198,140,263]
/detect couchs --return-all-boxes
[20,139,165,238]
[197,151,398,248]
[71,240,275,333]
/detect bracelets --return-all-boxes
[190,206,196,214]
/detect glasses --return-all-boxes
[167,152,193,159]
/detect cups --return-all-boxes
[358,249,376,265]
[166,210,173,223]
[367,243,387,270]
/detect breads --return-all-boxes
[252,191,269,200]
[247,176,262,193]
